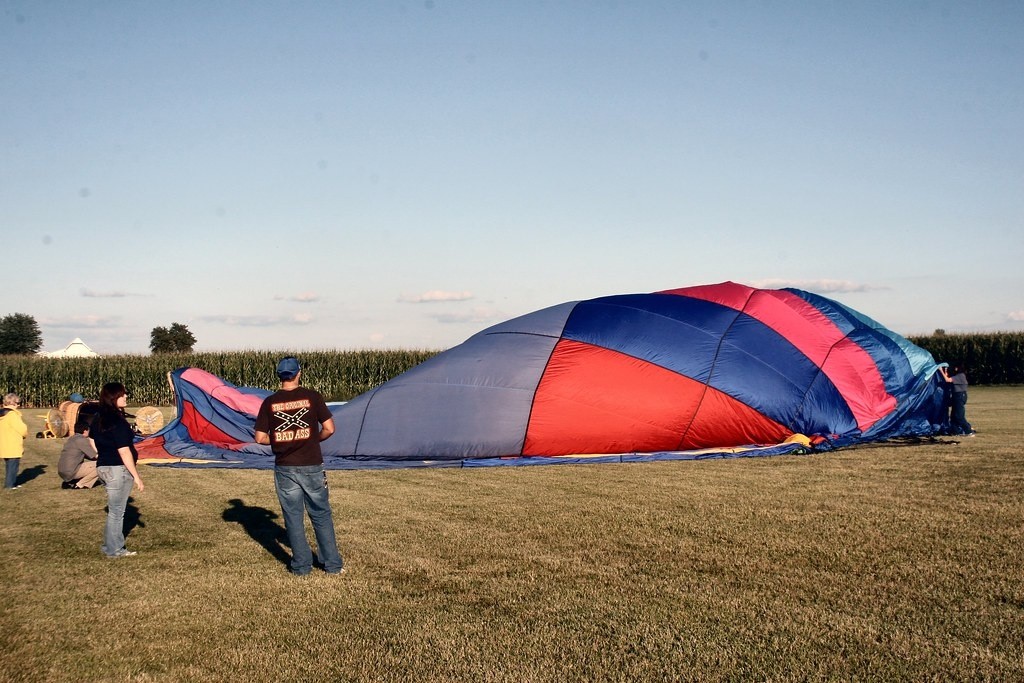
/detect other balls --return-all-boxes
[70,393,83,402]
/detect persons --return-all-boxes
[0,393,27,491]
[57,420,99,490]
[93,381,144,559]
[253,355,347,575]
[939,363,975,437]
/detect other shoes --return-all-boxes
[107,550,138,557]
[63,482,80,490]
[9,485,22,489]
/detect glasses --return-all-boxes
[15,401,21,406]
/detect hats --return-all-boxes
[276,357,301,379]
